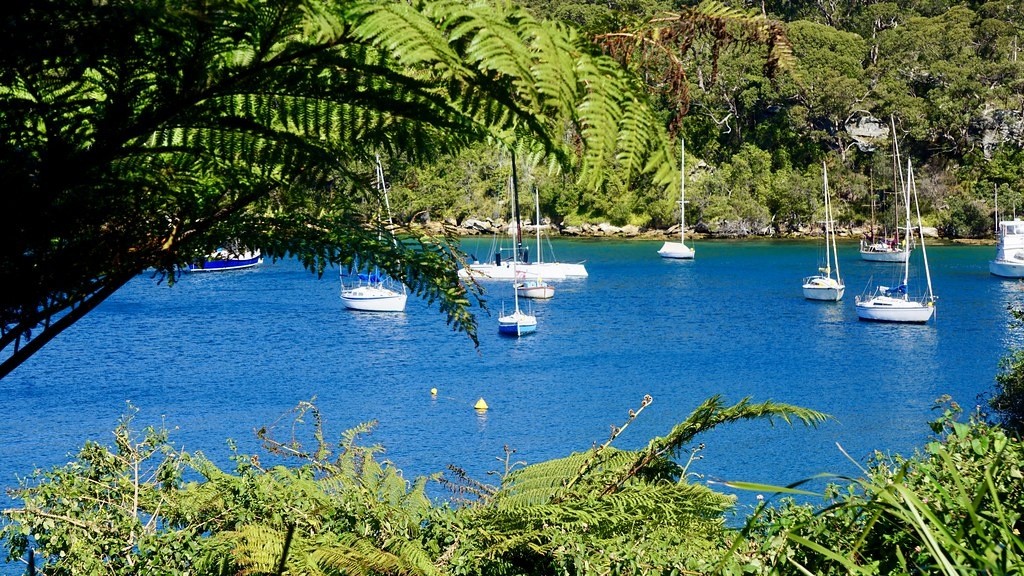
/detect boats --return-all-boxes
[498,176,537,336]
[189,248,264,272]
[513,188,555,299]
[989,182,1024,280]
[657,138,696,259]
[802,161,845,302]
[457,149,589,280]
[339,156,408,312]
[854,113,939,325]
